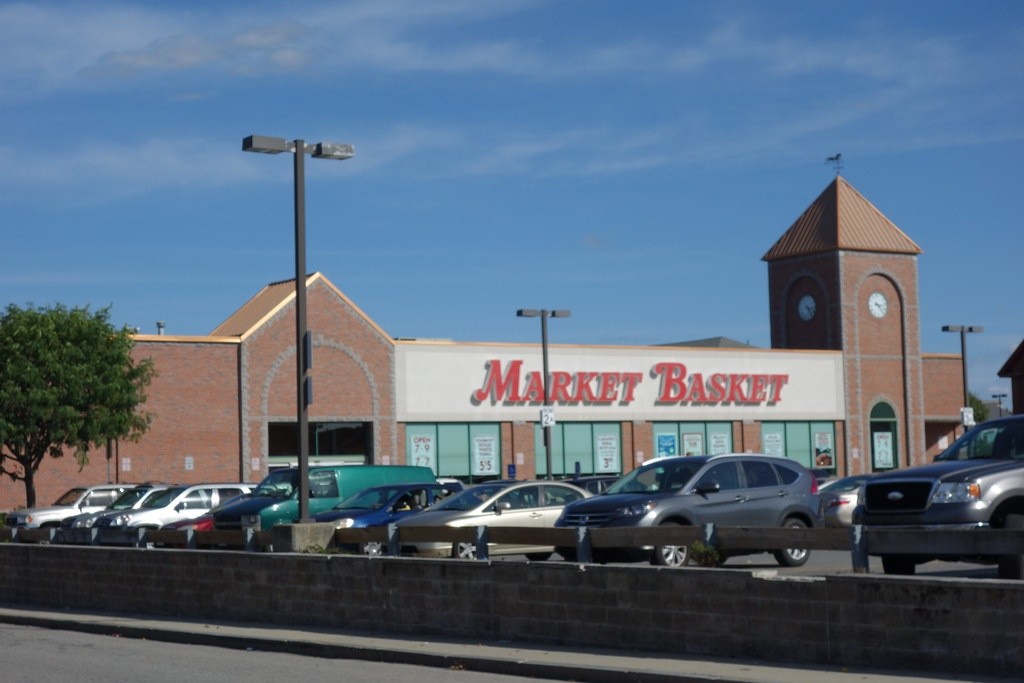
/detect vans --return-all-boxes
[193,463,443,551]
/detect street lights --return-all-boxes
[516,307,572,481]
[991,393,1009,418]
[941,324,987,432]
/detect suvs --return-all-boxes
[99,482,292,547]
[1,483,140,545]
[242,134,356,524]
[553,454,820,566]
[59,481,209,546]
[852,414,1024,580]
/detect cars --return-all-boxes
[396,479,593,561]
[314,484,494,555]
[567,476,650,496]
[819,474,878,527]
